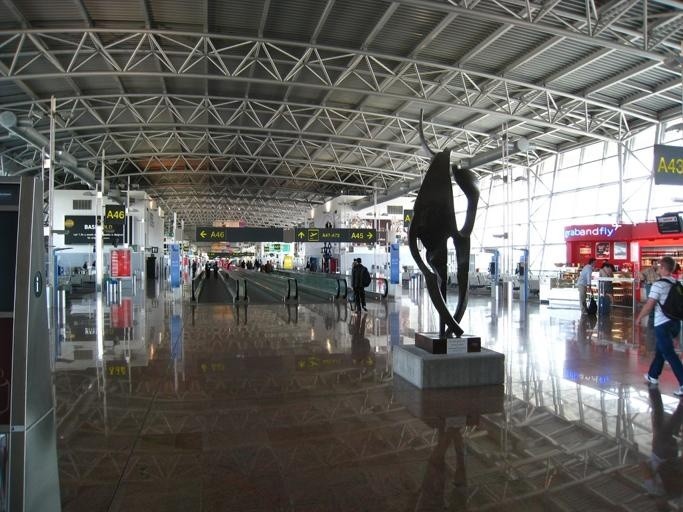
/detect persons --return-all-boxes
[577,258,596,315]
[305,261,317,272]
[598,262,614,305]
[636,257,683,396]
[240,259,273,275]
[351,311,370,363]
[644,379,683,511]
[600,258,615,272]
[351,258,369,313]
[640,258,662,328]
[192,260,196,278]
[205,260,219,281]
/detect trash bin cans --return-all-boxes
[495,281,512,304]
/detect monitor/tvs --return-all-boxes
[656,214,681,234]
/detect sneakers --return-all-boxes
[673,385,683,396]
[355,310,361,314]
[362,306,368,311]
[643,373,659,385]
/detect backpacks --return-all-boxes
[655,278,683,324]
[588,296,597,314]
[361,267,371,288]
